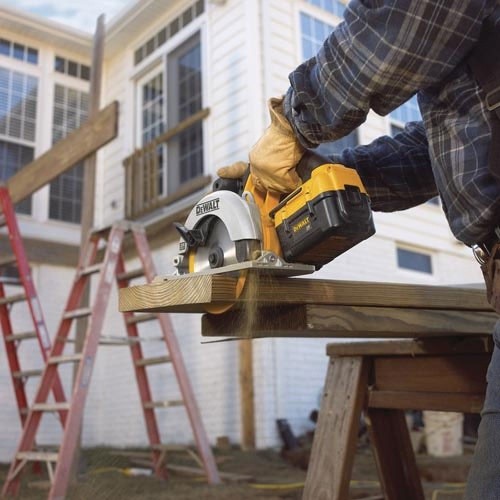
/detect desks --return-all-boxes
[300,335,492,500]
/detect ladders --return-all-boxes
[0,185,78,477]
[1,218,220,500]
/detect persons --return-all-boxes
[216,0,499,499]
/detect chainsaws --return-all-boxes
[169,148,378,317]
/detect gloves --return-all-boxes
[217,159,249,181]
[248,92,304,195]
